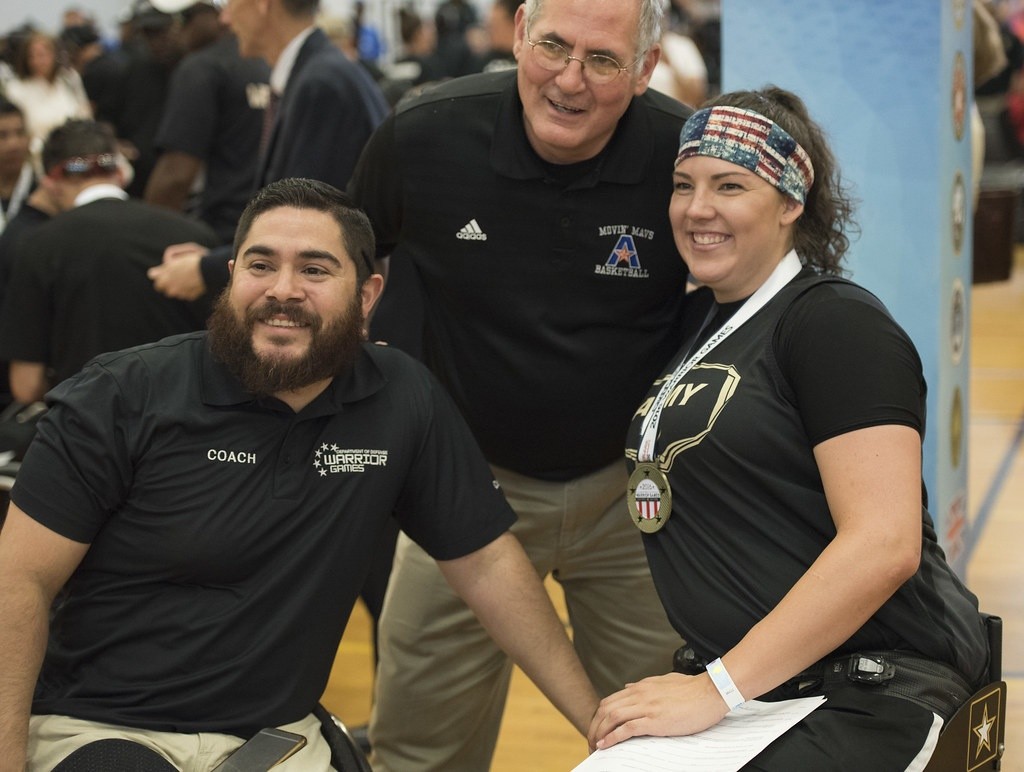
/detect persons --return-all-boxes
[0,119,222,536]
[0,0,274,234]
[571,84,989,772]
[395,0,720,113]
[351,2,698,772]
[0,178,599,772]
[147,0,393,336]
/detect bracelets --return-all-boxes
[705,658,745,711]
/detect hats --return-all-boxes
[43,136,134,185]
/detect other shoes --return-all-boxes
[347,723,373,753]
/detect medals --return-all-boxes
[627,458,672,533]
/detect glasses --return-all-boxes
[525,20,650,86]
